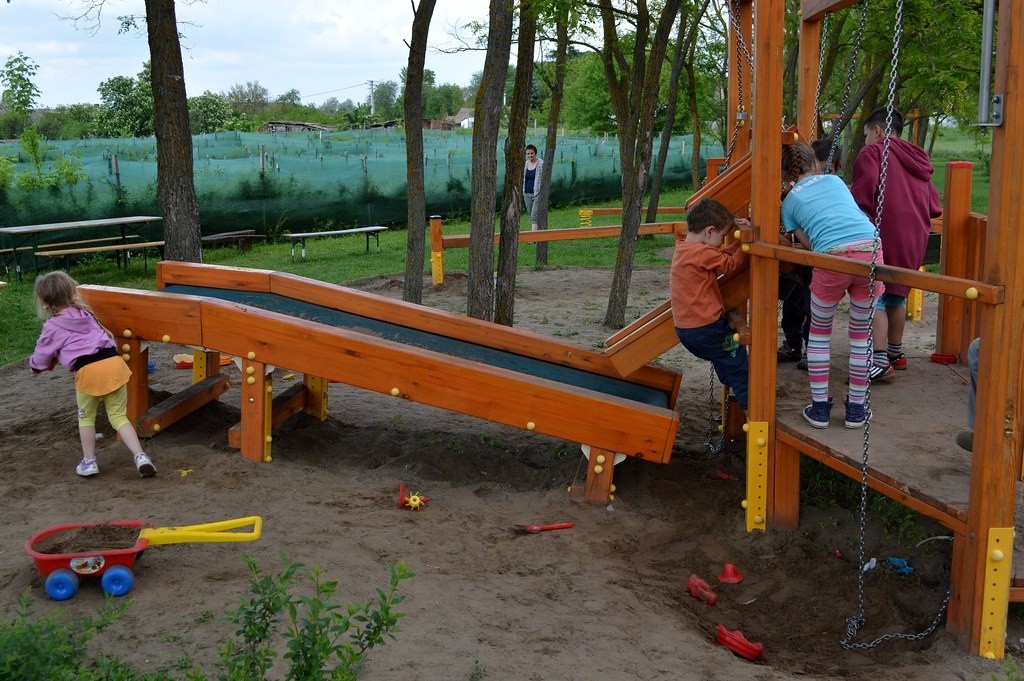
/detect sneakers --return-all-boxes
[76,456,99,476]
[845,360,896,383]
[886,353,907,369]
[134,453,157,478]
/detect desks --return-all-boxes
[0,216,165,283]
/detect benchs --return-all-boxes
[0,235,139,282]
[35,241,165,277]
[201,230,266,251]
[282,226,388,264]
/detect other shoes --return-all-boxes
[844,394,872,428]
[802,396,834,428]
[957,431,973,451]
[796,359,808,371]
[778,340,802,361]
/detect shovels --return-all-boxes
[510,522,574,535]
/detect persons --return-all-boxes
[670,198,754,417]
[956,337,979,452]
[776,141,843,372]
[29,271,157,477]
[781,141,873,428]
[523,144,544,244]
[846,107,943,384]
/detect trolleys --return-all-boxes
[24,515,263,600]
